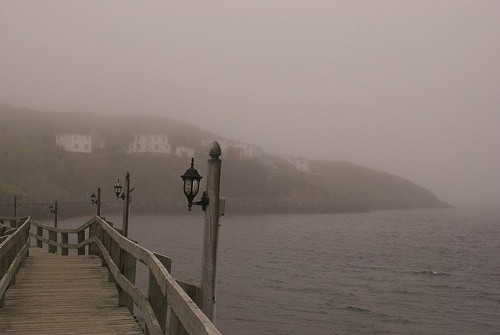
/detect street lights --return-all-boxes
[114,171,134,237]
[180,140,226,325]
[91,187,100,216]
[50,199,57,228]
